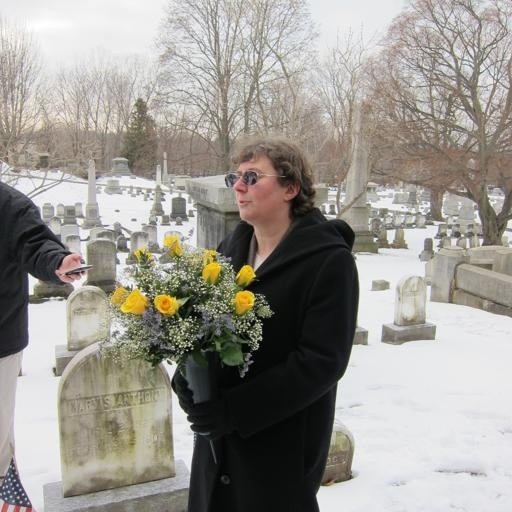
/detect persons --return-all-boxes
[169,134,363,511]
[1,182,88,511]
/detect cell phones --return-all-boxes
[65,265,93,275]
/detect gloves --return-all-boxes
[172,373,193,413]
[187,400,225,439]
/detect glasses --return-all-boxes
[225,171,286,188]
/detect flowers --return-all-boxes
[97,230,277,384]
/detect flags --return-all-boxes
[0,454,34,512]
